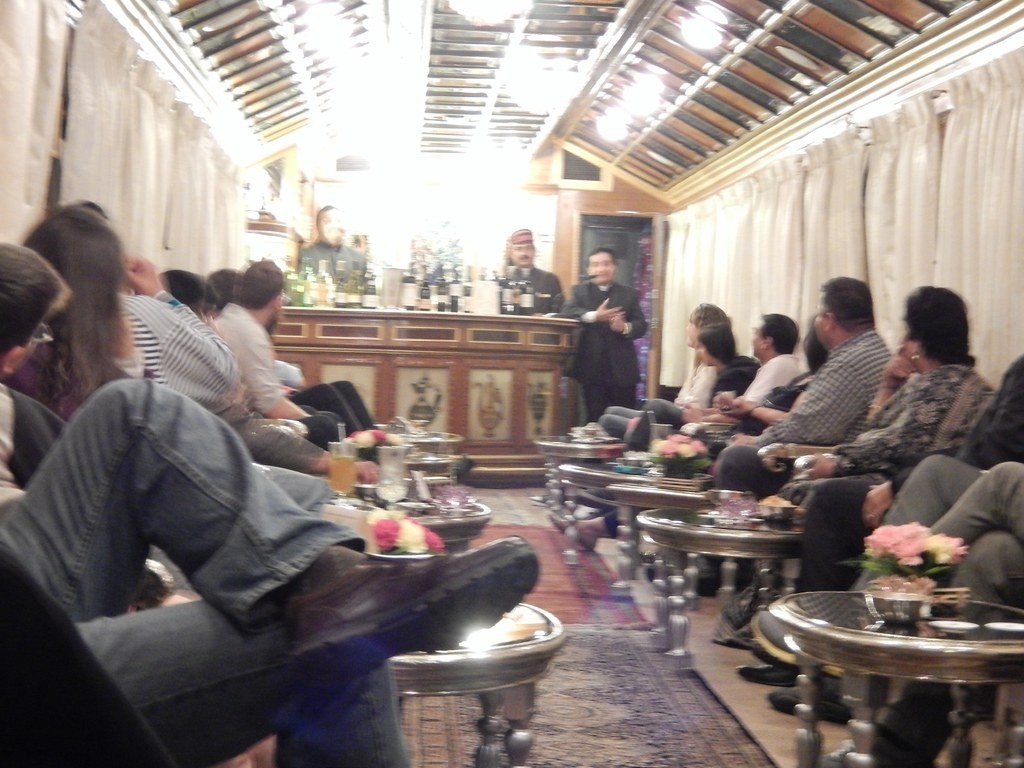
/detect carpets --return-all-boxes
[397,523,781,768]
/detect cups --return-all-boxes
[719,390,735,411]
[651,424,673,441]
[338,424,347,442]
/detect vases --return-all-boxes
[665,462,692,479]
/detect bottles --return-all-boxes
[316,259,349,308]
[420,279,431,311]
[435,271,473,314]
[495,265,534,316]
[349,260,380,308]
[282,255,317,308]
[401,276,417,310]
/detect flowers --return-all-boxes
[840,520,969,594]
[366,509,444,557]
[650,433,710,470]
[348,429,401,447]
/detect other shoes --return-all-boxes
[770,669,852,725]
[735,657,801,686]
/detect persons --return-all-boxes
[505,227,567,315]
[558,222,1024,768]
[0,199,539,768]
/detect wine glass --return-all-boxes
[328,443,357,510]
[376,445,412,511]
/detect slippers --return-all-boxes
[548,514,595,552]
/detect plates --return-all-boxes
[928,621,979,634]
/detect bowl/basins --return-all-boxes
[755,504,798,521]
[616,458,649,466]
[584,429,599,435]
[865,593,928,625]
[353,484,377,501]
[708,490,744,505]
[376,425,402,433]
[411,420,430,429]
[437,485,476,505]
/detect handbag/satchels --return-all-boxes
[712,586,783,653]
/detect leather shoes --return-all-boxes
[289,535,540,682]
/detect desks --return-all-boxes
[554,462,659,565]
[339,431,565,768]
[638,508,806,669]
[758,590,1023,767]
[536,432,629,509]
[605,481,716,607]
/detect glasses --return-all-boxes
[31,322,54,344]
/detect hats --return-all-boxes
[512,229,532,244]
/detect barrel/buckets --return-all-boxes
[473,281,500,316]
[382,267,404,308]
